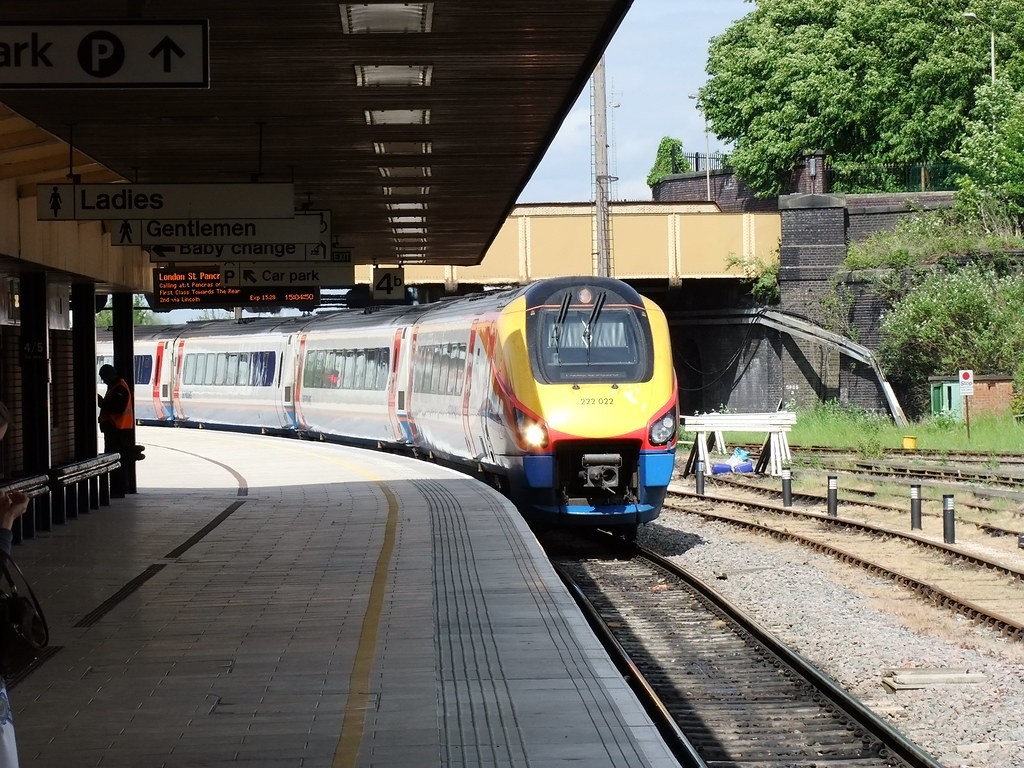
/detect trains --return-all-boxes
[92,274,681,540]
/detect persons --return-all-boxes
[0,402,30,768]
[98,364,134,498]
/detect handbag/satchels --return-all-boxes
[0,549,49,677]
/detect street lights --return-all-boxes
[962,11,1001,196]
[685,92,713,201]
